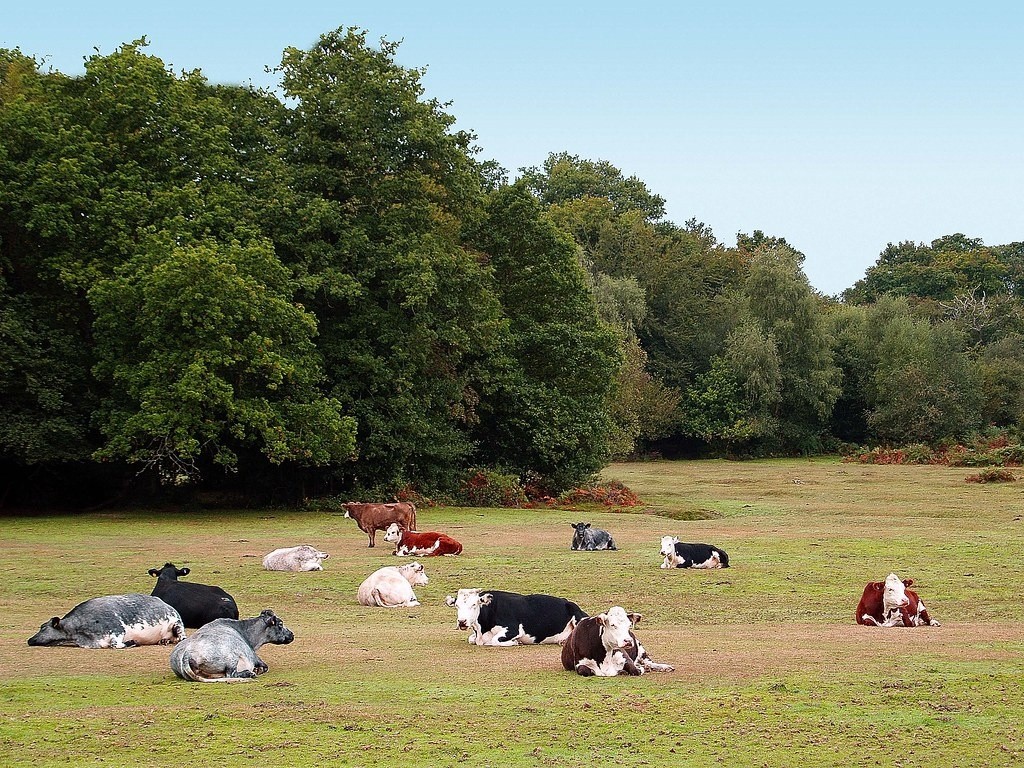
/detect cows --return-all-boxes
[169,609,295,684]
[855,572,942,627]
[356,560,431,608]
[569,521,617,551]
[26,593,187,653]
[341,501,417,548]
[357,560,431,609]
[382,522,464,558]
[444,586,589,648]
[261,544,330,572]
[561,605,675,678]
[147,561,240,630]
[658,535,731,570]
